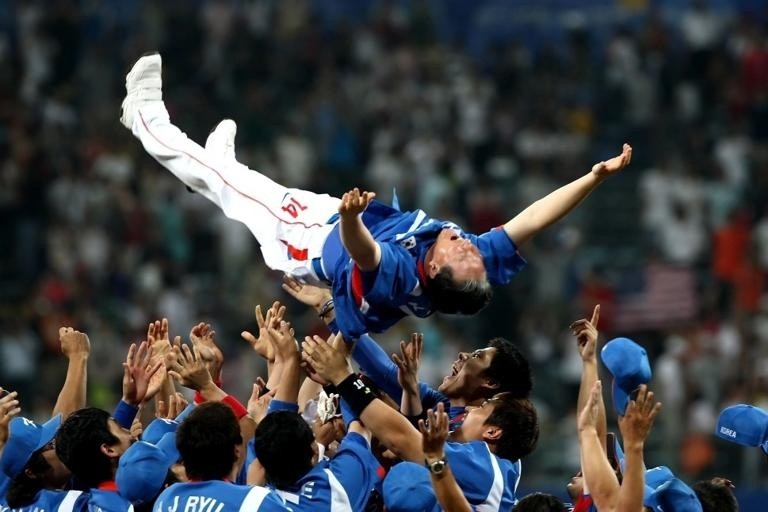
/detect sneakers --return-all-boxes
[120,51,164,129]
[205,118,237,163]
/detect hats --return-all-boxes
[116,403,198,505]
[0,414,62,477]
[600,337,653,416]
[382,461,438,510]
[716,404,768,454]
[607,432,702,512]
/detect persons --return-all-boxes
[118,50,632,342]
[0,277,739,510]
